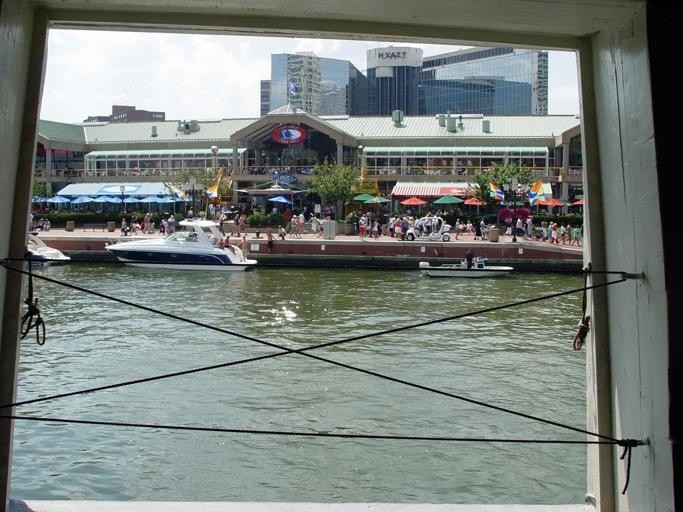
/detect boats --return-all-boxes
[105,220,258,272]
[419,257,515,278]
[28,232,71,264]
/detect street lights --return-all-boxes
[185,177,204,218]
[503,176,526,242]
[120,184,126,219]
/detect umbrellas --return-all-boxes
[353,193,373,201]
[123,196,141,203]
[539,198,565,206]
[572,199,583,205]
[433,195,464,204]
[138,196,162,212]
[175,195,194,212]
[157,195,181,202]
[91,196,111,202]
[45,195,70,203]
[106,196,122,203]
[70,195,93,203]
[363,196,392,203]
[463,197,488,206]
[267,195,296,205]
[31,195,47,203]
[400,196,428,205]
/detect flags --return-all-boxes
[526,179,546,206]
[204,184,219,198]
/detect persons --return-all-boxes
[38,218,44,231]
[120,211,176,236]
[236,236,248,262]
[187,206,194,215]
[266,229,276,252]
[224,235,235,253]
[288,81,298,95]
[216,211,224,236]
[230,213,239,236]
[234,216,242,237]
[217,239,224,250]
[184,234,197,241]
[356,207,584,249]
[29,213,34,224]
[209,204,217,213]
[277,224,287,239]
[292,216,304,238]
[308,214,320,234]
[299,210,308,227]
[43,219,51,231]
[464,247,474,268]
[289,215,299,239]
